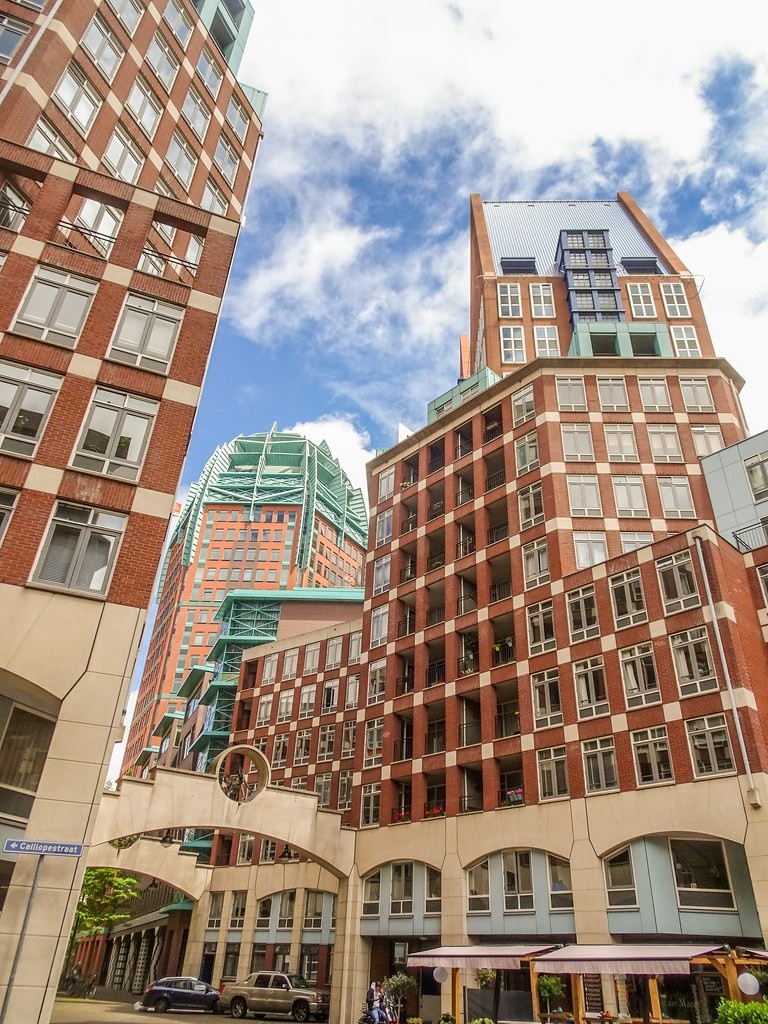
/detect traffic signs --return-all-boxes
[3,839,84,858]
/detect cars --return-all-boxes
[141,976,228,1014]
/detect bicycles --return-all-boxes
[61,969,100,1000]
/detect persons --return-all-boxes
[366,982,387,1024]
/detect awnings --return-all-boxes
[406,945,557,970]
[748,948,767,957]
[529,944,723,975]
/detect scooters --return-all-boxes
[357,994,400,1024]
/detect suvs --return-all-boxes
[218,970,330,1022]
